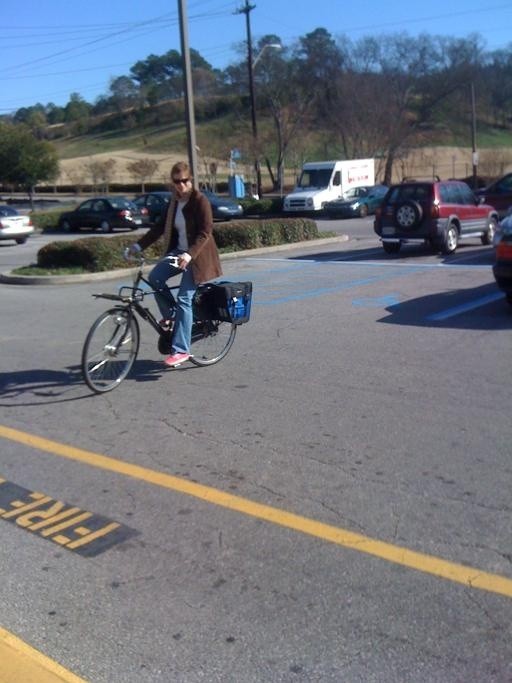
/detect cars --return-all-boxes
[132,192,172,225]
[323,185,390,220]
[0,204,36,244]
[57,197,149,234]
[199,189,244,222]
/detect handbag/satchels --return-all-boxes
[213,281,251,323]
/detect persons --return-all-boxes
[123,162,223,366]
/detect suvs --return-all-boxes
[373,176,499,254]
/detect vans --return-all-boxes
[283,157,375,219]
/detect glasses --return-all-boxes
[172,177,188,184]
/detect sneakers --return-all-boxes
[159,318,175,325]
[163,352,189,365]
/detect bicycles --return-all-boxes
[80,242,238,394]
